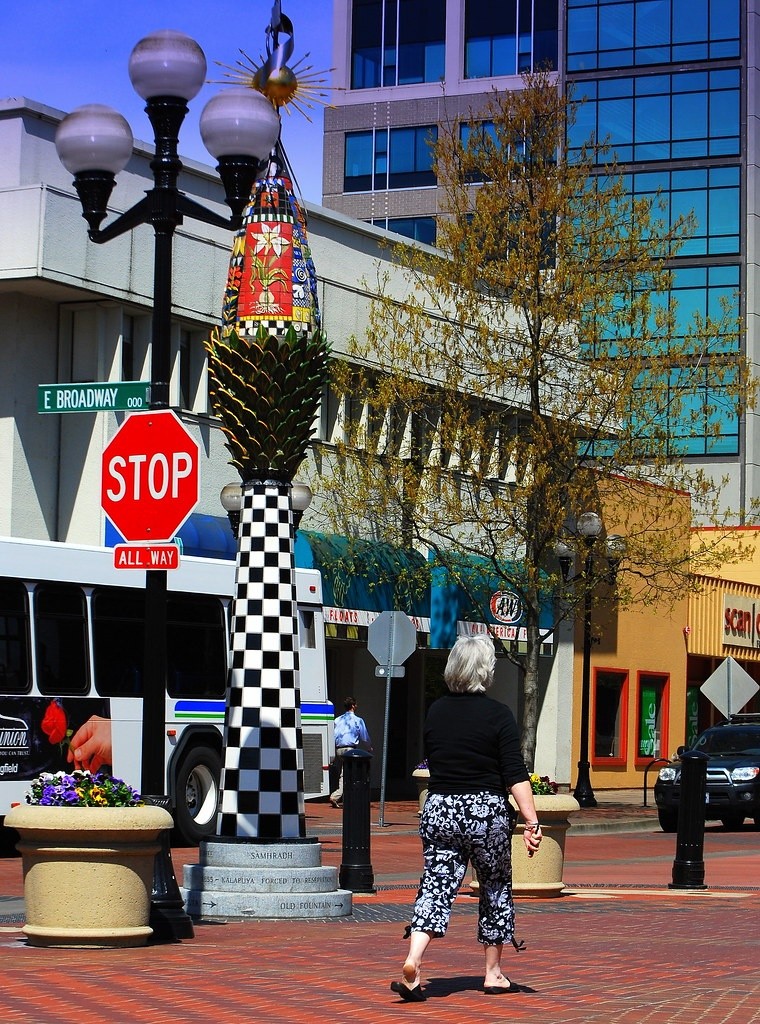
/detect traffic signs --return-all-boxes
[35,382,151,414]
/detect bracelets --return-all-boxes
[525,822,538,825]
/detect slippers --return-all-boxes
[484,976,520,995]
[390,981,426,1002]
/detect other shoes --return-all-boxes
[329,796,340,808]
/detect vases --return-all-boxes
[4,804,174,949]
[469,793,580,898]
[412,770,431,818]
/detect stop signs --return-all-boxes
[103,411,200,545]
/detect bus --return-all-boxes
[1,538,337,849]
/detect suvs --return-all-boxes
[653,712,760,834]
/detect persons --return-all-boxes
[329,696,373,806]
[390,635,542,1000]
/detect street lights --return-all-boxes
[551,512,628,810]
[49,31,280,945]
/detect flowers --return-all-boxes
[40,699,87,771]
[24,770,146,808]
[415,758,429,770]
[528,772,559,796]
